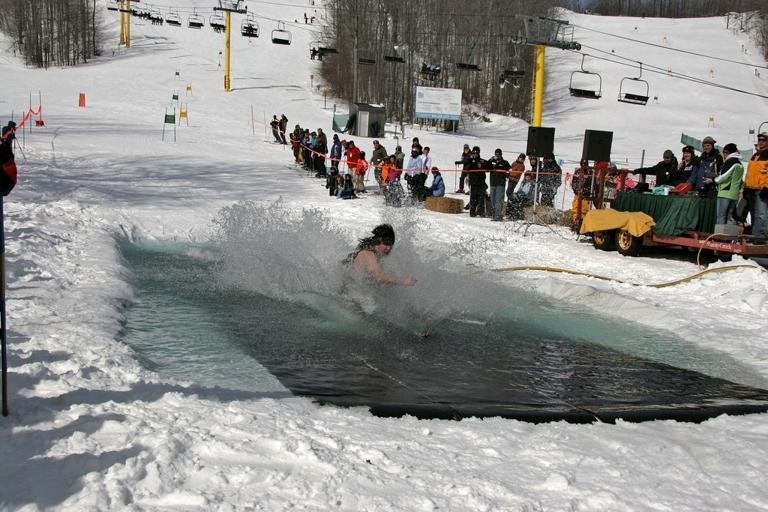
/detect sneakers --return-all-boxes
[455,189,470,195]
[478,207,486,218]
[469,206,477,217]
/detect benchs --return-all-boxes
[425,196,464,215]
[502,201,556,224]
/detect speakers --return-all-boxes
[526,127,555,158]
[581,129,613,162]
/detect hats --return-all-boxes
[431,167,439,174]
[463,143,557,161]
[702,136,716,149]
[757,131,768,139]
[723,143,738,154]
[682,145,695,160]
[373,140,419,155]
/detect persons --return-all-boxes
[340,223,417,300]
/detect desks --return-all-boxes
[613,189,715,237]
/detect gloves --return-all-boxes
[743,188,752,200]
[759,186,768,202]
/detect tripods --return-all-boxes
[10,133,28,163]
[515,157,557,237]
